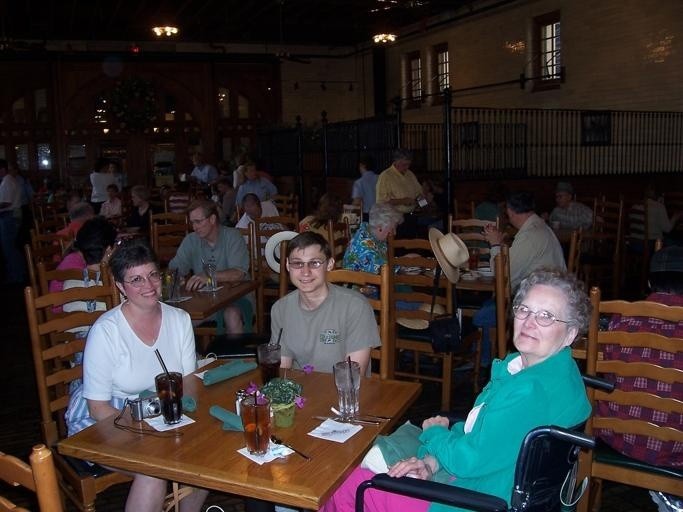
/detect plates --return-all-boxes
[402,266,426,276]
[462,267,494,280]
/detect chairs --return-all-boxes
[0,440,61,512]
[575,285,682,512]
[22,277,134,511]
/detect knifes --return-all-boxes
[310,415,381,427]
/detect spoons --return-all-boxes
[267,432,315,462]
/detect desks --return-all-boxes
[56,359,423,511]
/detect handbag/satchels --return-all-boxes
[429,317,462,353]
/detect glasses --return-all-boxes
[122,270,161,289]
[288,257,329,269]
[510,302,568,327]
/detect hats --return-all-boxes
[428,228,471,285]
[265,231,301,274]
[395,302,445,331]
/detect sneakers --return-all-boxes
[648,490,683,512]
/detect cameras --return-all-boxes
[130,396,162,422]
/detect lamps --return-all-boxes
[373,3,398,43]
[150,5,181,38]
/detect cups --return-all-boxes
[255,341,281,385]
[162,262,216,300]
[154,371,184,426]
[239,394,272,457]
[332,360,360,415]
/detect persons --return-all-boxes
[299,193,444,314]
[540,180,603,250]
[2,159,33,277]
[629,184,680,273]
[66,240,218,512]
[475,184,506,258]
[351,148,442,239]
[308,263,595,512]
[453,191,566,379]
[50,151,286,379]
